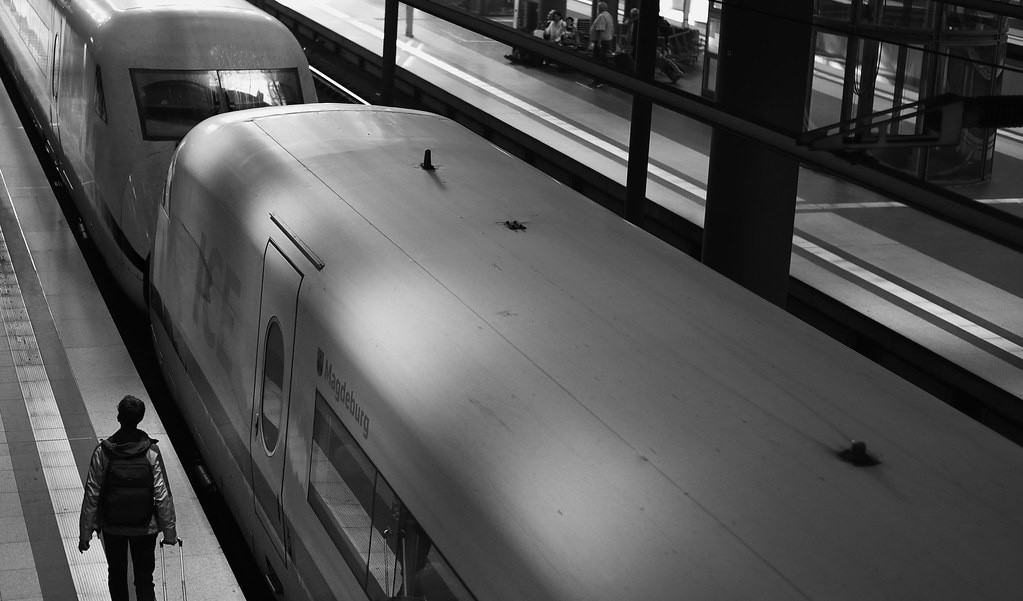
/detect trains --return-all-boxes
[150,103,1023,601]
[0,0,317,317]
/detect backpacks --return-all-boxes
[97,457,154,527]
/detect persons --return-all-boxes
[588,1,614,88]
[79,395,178,601]
[626,7,673,68]
[504,11,579,64]
